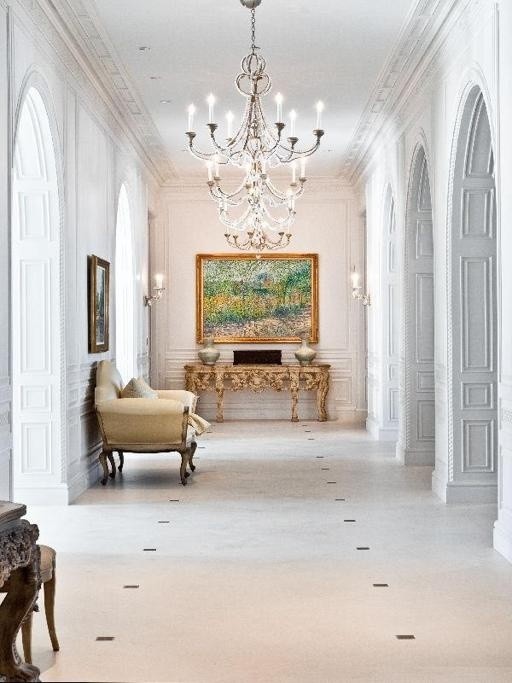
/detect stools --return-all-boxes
[0,544,59,664]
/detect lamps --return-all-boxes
[350,272,371,310]
[144,274,165,307]
[186,0,325,252]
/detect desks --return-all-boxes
[0,501,42,683]
[184,363,331,423]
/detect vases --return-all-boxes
[293,340,317,366]
[198,339,220,365]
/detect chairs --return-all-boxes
[94,360,211,486]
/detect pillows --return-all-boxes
[120,377,158,398]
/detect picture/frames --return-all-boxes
[195,253,319,345]
[89,254,110,353]
